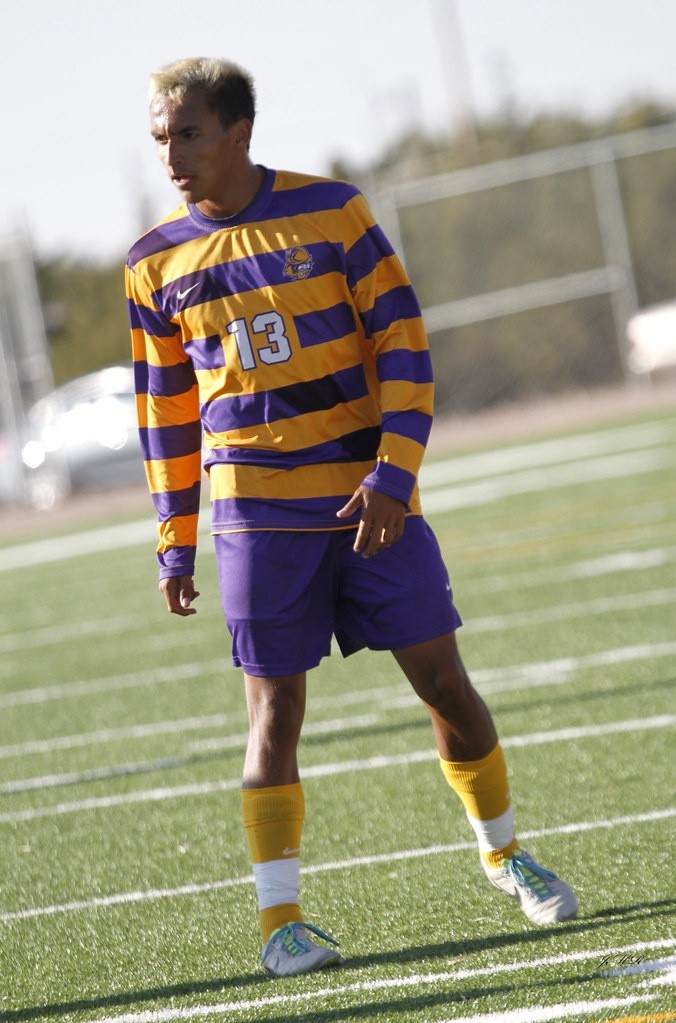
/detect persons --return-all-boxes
[123,56,577,976]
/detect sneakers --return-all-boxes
[261,921,342,976]
[479,852,579,925]
